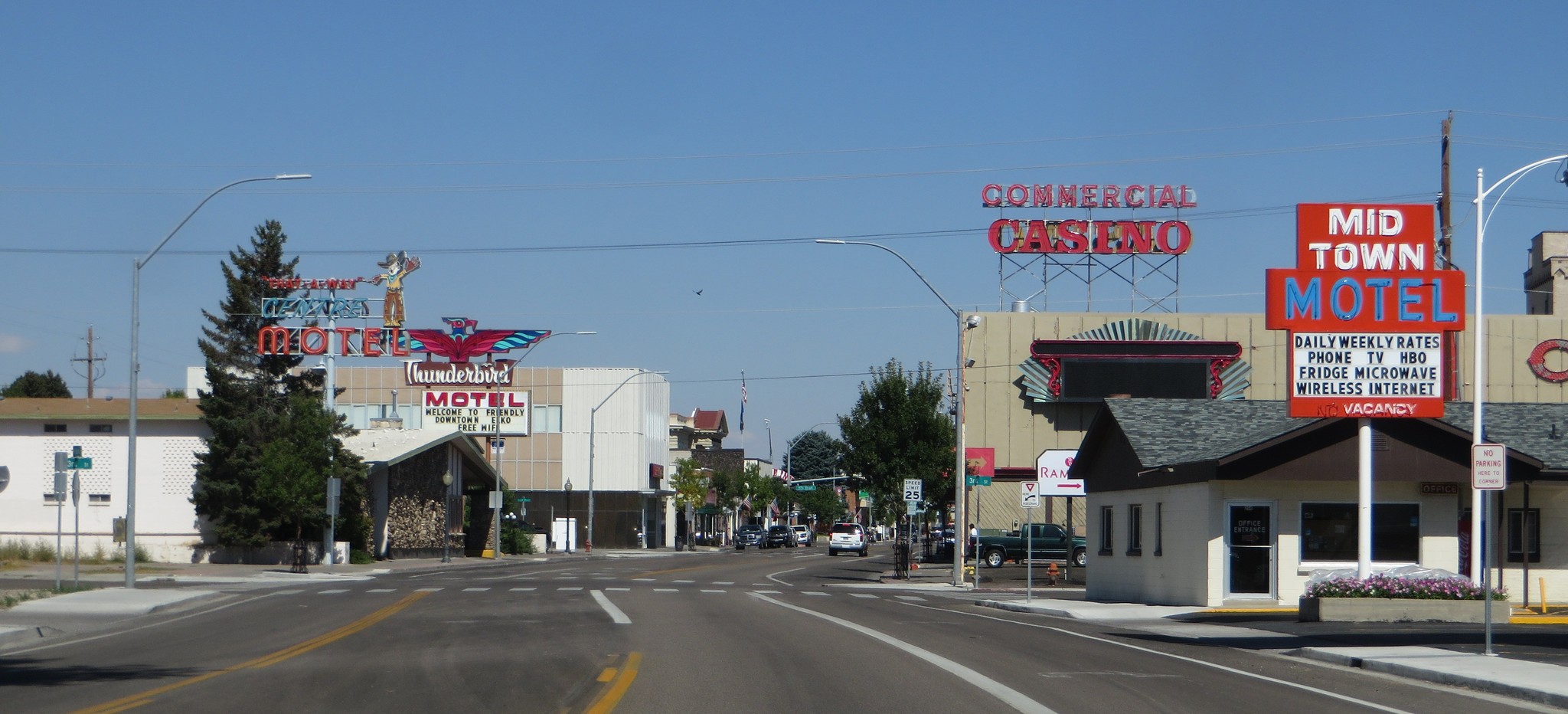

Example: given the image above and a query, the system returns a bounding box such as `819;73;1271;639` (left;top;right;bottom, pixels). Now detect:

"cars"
930;524;955;554
790;528;798;547
511;520;551;552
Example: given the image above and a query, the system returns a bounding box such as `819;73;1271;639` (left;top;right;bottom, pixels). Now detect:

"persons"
969;523;977;537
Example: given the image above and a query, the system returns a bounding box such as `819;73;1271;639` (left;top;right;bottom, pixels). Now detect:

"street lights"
564;477;572;554
495;331;598;559
816;237;981;584
441;470;453;564
588;370;670;551
124;173;314;589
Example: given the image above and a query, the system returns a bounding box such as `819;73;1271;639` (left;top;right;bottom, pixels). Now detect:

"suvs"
791;524;812;547
894;524;919;542
767;525;793;549
828;522;871;557
733;524;768;550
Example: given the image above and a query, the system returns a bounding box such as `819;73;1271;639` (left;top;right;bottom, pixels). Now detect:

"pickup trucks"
969;522;1087;568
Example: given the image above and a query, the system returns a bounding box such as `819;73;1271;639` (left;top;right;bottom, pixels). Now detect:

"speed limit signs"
903;478;922;501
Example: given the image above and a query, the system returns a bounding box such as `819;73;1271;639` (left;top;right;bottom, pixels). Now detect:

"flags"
744;496;751;511
770;499;780;516
858;510;862;523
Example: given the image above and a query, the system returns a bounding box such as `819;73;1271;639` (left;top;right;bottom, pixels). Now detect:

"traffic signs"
1019;481;1040;508
1035;449;1086;497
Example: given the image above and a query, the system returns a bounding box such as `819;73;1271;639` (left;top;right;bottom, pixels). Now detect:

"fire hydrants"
585;539;592;553
1046;562;1060;586
